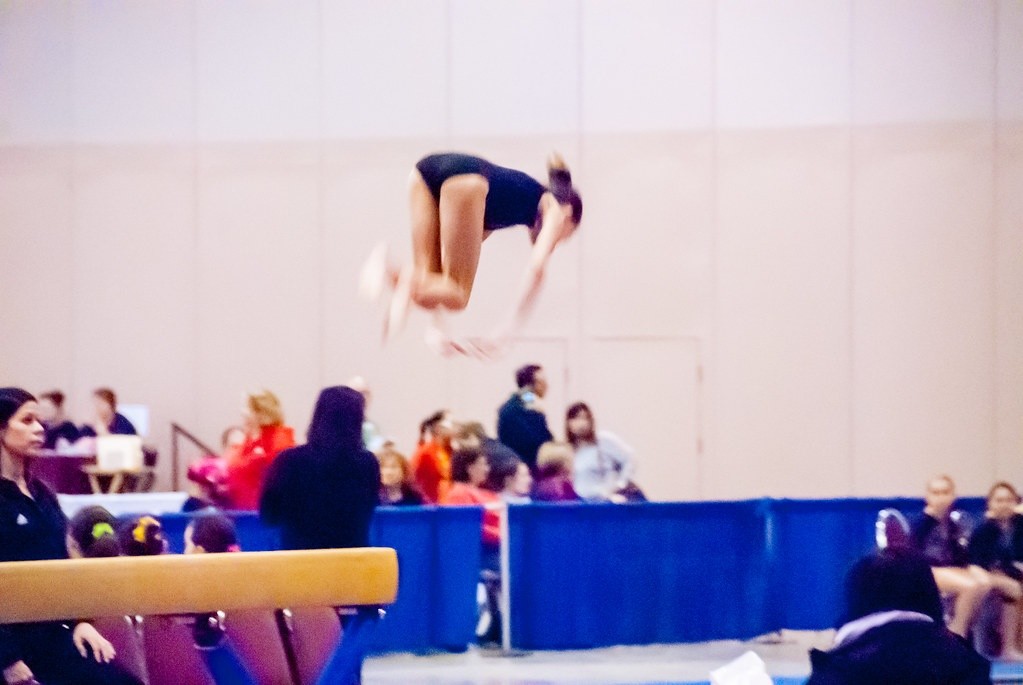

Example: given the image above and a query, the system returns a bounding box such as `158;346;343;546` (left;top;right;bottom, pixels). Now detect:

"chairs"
878;506;1023;656
86;610;345;685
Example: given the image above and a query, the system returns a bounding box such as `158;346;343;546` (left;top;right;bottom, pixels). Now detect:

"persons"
968;484;1023;660
0;387;144;685
79;390;136;437
415;411;453;500
498;364;555;463
374;447;428;506
120;515;168;555
36;392;79;448
183;515;237;552
63;506;121;558
560;402;631;502
258;385;381;551
531;440;575;502
805;547;992;685
360;152;583;361
221;392;295;511
449;451;502;570
490;456;533;500
453;411;531;492
910;474;990;638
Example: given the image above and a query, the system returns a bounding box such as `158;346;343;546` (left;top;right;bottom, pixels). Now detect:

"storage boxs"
92;433;145;471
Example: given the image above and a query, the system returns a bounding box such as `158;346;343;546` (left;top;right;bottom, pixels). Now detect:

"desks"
82;467;158;491
30;454;157;492
